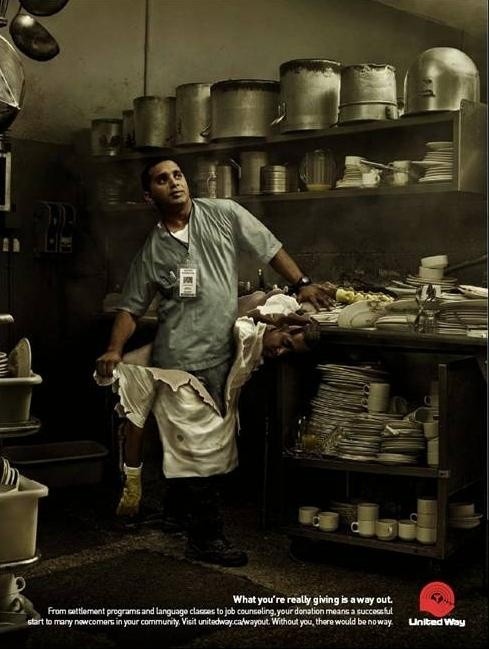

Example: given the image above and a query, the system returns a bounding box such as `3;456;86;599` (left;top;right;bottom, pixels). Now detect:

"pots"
260;164;291;194
189;153;238;198
9;13;61;63
268;58;341;135
402;44;481;120
200;78;278;143
328;63;398;127
122;110;134;152
91;118;122;157
175;82;210;148
230;150;269;198
133;95;177;149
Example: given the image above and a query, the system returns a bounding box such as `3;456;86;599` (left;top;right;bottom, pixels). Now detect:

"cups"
393;518;416;543
361;381;391;414
349;518;377;540
407;382;439;467
356;501;379;520
414;515;438;531
361;173;381;189
297;505;319;525
313;510;339;534
416;497;438;516
386;158;410;184
1;571;28;613
413;526;440;547
375;518;399;541
299;148;332;194
450;501;476;515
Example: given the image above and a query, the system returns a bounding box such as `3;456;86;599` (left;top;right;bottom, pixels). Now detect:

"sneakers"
114;477;143;519
186;537;247;567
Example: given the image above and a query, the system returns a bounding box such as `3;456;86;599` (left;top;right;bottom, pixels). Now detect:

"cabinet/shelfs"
65;100;487;212
270;328;487;578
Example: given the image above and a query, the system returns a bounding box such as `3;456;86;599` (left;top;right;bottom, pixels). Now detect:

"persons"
93;157;336;565
114;294;321;516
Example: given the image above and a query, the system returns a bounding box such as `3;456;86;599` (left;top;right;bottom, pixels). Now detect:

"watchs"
294;275;311;290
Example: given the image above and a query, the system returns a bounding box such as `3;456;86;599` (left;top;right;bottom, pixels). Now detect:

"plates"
410;140;456;183
299;363;426;465
309;254;486;340
1;458;22;494
337;298;382;328
454;515;483;530
335;156;367;190
0;347;10;376
12;334;34;378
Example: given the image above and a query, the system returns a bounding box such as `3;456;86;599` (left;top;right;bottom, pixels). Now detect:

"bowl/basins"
419;255;449;279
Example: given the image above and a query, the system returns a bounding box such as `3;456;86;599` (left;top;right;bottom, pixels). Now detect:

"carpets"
0;495;489;649
